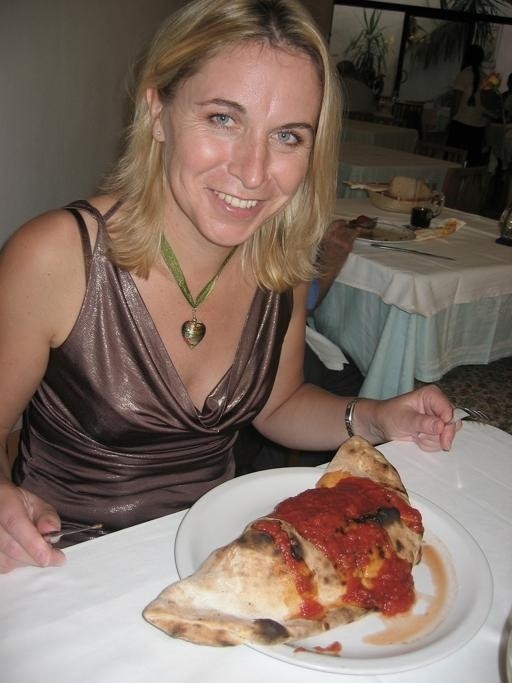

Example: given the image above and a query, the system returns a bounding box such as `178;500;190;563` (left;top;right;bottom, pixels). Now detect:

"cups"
410;176;446;229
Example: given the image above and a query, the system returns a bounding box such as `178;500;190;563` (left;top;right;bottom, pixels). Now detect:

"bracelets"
344;395;367;438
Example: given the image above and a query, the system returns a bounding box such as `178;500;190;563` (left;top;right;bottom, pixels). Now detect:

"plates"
173;466;495;676
345;220;417;244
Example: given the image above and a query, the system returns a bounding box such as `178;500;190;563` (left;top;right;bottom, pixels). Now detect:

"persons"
1;0;462;576
303;216;361;393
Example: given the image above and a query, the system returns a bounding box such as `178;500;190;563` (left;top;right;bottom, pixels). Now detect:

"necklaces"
154;236;243;350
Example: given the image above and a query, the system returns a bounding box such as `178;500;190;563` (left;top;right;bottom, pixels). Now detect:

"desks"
334;119;465;199
308;199;511;401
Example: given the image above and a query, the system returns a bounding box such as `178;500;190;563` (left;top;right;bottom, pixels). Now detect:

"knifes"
371;244;457;261
42;524;104;540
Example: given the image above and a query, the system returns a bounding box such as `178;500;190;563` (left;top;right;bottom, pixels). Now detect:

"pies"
143;435;424;649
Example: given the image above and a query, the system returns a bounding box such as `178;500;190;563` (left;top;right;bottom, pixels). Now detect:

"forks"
445;406;492;427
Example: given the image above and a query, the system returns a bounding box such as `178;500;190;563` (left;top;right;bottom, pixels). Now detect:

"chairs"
386;101;423;130
